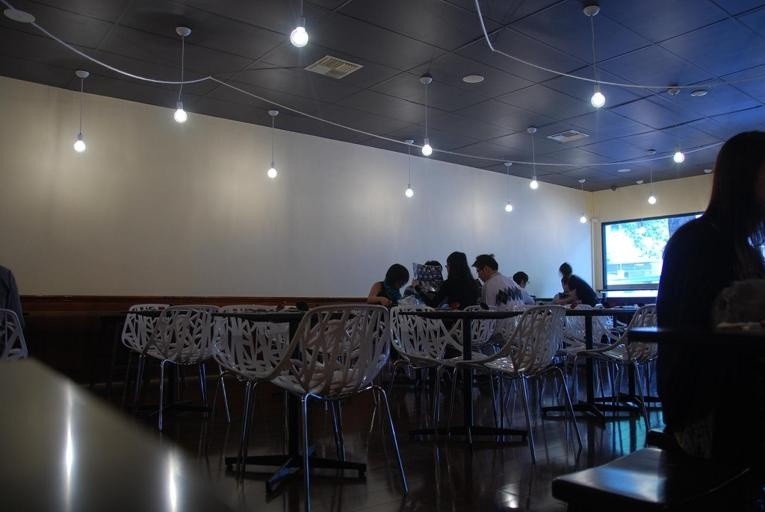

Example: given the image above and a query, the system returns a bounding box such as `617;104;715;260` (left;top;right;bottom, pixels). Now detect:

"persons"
655;129;764;511
366;251;598;396
